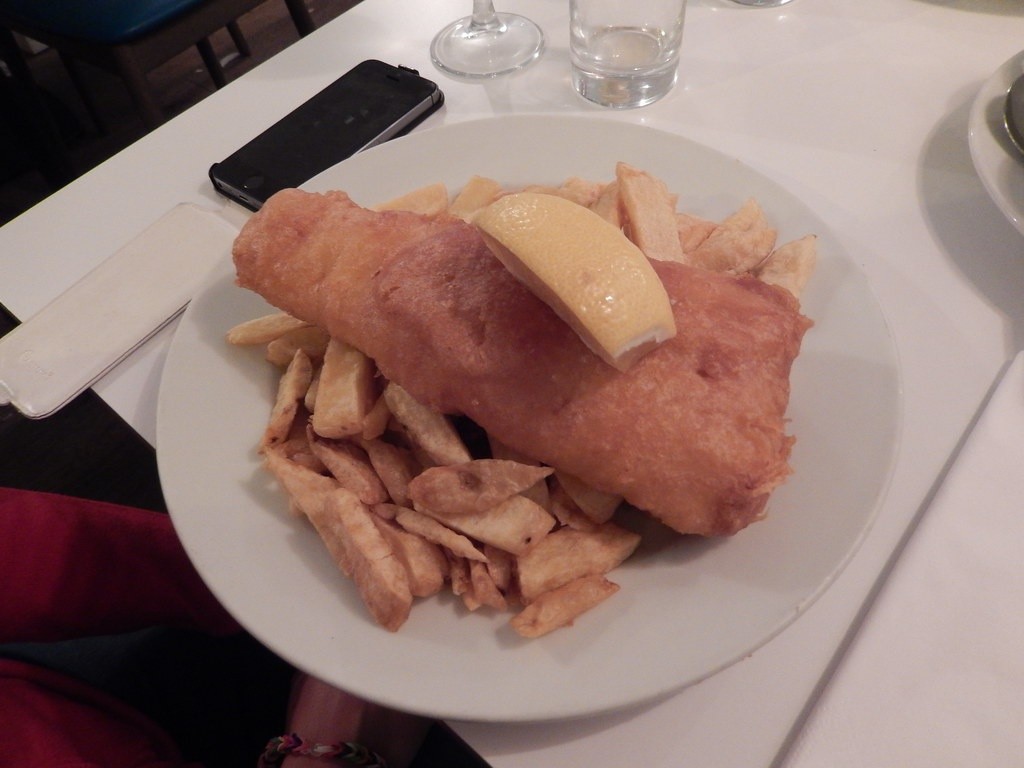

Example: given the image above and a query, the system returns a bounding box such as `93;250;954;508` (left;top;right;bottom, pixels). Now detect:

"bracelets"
257;735;391;768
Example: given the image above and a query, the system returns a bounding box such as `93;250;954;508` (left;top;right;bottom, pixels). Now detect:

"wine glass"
430;0;545;80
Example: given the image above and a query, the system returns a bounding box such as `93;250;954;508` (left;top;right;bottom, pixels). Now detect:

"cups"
568;0;687;111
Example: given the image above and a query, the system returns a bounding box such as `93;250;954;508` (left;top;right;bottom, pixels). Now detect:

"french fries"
226;160;818;644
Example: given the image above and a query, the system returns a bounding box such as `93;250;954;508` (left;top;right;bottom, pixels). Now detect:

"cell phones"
209;60;443;213
1;201;240;422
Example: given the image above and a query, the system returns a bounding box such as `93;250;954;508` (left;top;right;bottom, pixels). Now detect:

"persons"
0;485;431;768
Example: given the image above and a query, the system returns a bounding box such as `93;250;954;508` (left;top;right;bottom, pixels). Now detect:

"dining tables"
0;0;1024;767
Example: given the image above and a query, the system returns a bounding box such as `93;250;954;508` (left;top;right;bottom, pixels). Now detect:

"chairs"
0;0;317;135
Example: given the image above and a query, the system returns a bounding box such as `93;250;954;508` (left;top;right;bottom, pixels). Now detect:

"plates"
967;49;1024;238
156;111;907;722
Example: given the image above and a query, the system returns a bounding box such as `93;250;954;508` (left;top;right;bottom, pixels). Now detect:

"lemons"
471;191;677;370
